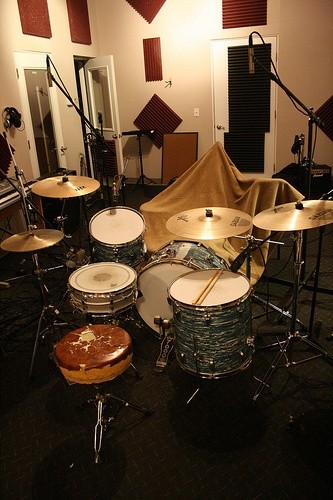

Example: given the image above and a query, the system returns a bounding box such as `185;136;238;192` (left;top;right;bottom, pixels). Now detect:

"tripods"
0;116;333;465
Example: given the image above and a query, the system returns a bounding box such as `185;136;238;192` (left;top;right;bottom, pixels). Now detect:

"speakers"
272;163;302;196
299;162;332;200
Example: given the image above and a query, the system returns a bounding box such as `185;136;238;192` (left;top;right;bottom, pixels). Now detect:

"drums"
167;269;255;378
136;241;230;337
89;206;146;267
69;262;137;318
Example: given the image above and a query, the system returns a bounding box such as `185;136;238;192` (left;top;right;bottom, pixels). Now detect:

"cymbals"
166;207;252;239
252;200;333;231
31;175;100;198
1;229;64;252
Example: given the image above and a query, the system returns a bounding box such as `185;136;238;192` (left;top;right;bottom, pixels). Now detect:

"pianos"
0;176;37;211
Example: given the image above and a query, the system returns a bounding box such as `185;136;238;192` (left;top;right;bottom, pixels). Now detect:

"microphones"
247;34;256;74
47;55;52;87
291;134;305;154
56;256;76;269
